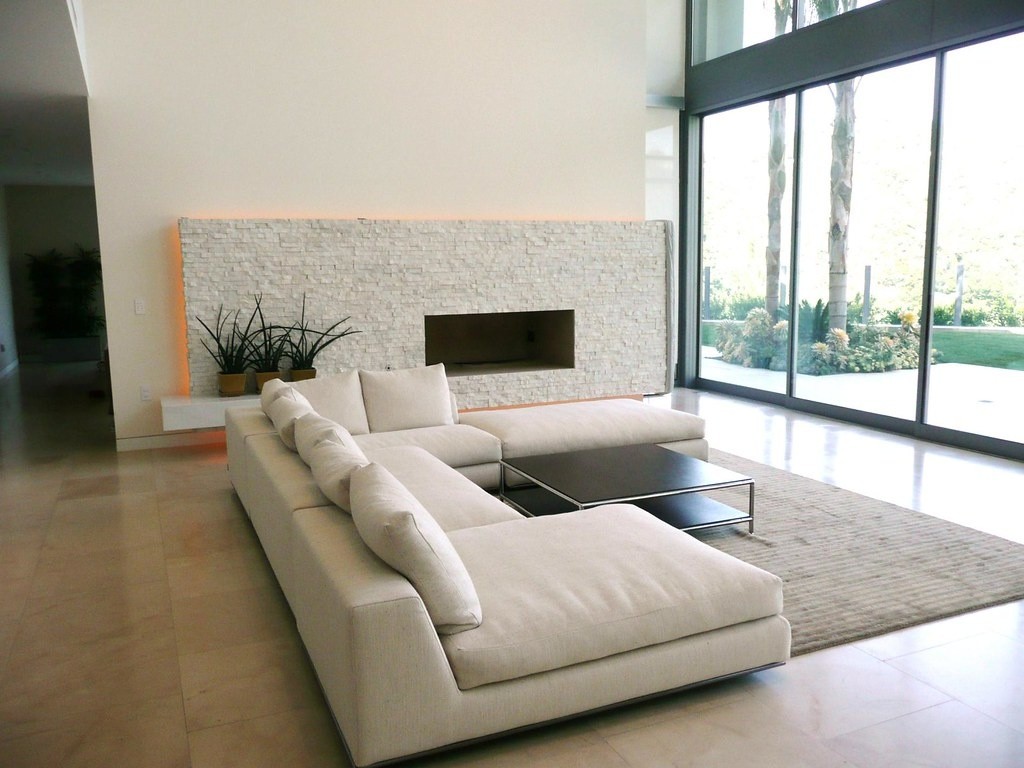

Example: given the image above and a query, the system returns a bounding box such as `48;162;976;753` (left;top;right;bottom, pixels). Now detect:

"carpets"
493;447;1024;661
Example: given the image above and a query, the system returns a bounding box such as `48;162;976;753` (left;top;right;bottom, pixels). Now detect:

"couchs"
224;361;794;768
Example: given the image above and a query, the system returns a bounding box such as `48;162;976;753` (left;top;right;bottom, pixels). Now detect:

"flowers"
713;304;941;376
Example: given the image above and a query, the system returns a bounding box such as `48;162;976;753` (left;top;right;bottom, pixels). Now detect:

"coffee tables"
499;444;755;539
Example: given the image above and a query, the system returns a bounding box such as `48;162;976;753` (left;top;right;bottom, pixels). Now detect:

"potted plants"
280;291;363;381
18;242;106;363
231;292;299;395
194;292;263;398
